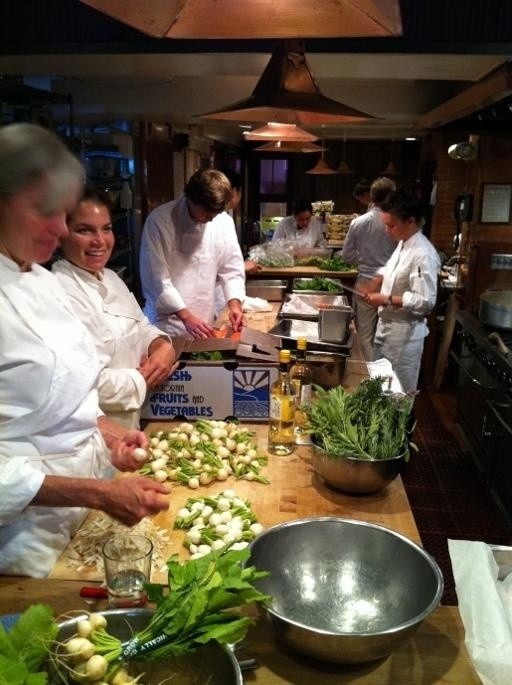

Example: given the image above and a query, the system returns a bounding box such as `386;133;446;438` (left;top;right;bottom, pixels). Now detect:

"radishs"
35;550;275;685
133;419;264;489
176;490;265;560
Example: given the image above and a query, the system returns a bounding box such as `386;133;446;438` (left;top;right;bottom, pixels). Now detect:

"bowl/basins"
248;518;446;669
51;611;244;685
307;432;406;496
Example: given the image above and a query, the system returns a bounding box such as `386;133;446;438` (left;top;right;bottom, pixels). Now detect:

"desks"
47;298;427;585
249;263;362;279
1;572;479;685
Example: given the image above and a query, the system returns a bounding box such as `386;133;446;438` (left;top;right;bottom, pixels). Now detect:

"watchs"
386;295;392;305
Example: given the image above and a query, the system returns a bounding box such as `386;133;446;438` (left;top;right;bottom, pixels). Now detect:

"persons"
272;199;326;252
224;172;263;275
0;122;172;579
138;169;247;339
357;193;442;415
352;178;370;205
50;196;176;430
340;176;397;361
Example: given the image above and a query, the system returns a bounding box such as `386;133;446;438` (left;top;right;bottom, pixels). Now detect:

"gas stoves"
453;306;512;376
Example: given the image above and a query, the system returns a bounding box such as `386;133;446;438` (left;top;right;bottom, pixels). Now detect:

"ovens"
444;332;511;525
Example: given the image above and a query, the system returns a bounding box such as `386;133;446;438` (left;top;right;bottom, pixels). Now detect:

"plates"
443;280;456;284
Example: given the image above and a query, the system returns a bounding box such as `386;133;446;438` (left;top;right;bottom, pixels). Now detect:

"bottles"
268;350;296;456
289;338;312;436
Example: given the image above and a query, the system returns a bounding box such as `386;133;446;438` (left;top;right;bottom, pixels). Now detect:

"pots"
478;287;512;329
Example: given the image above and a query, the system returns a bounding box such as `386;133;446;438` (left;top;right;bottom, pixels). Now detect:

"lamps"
304;136;338;174
251;142;330;153
191;51;386;125
240;122;322;143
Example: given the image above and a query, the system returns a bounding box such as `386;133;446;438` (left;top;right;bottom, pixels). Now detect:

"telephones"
454;192;472;222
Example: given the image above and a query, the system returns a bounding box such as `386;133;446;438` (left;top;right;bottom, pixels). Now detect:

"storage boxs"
138;327;283;424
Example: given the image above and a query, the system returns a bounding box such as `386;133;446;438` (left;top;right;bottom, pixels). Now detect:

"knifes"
324;277;367;298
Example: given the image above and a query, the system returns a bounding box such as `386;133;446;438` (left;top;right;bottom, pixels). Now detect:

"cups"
102;535;154;609
489;253;512;269
